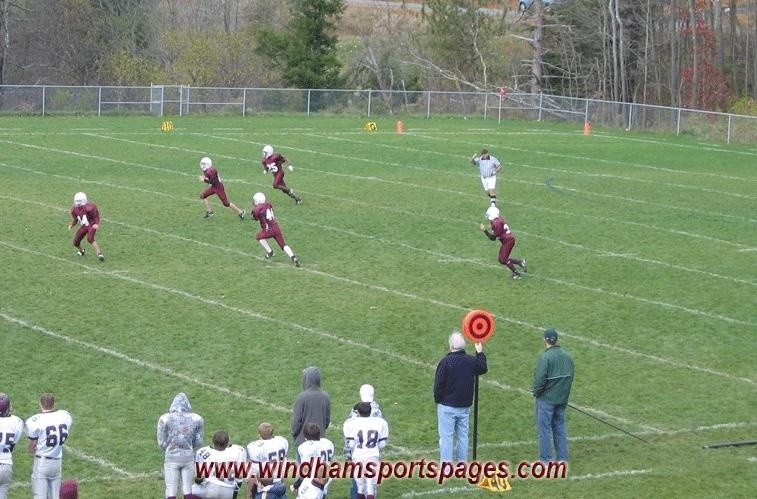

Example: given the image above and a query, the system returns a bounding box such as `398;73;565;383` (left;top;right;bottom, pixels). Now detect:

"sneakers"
73;248;87;258
202;209;216;220
96;252;105;262
511;270;522;280
520;258;528;273
293;257;301;268
287;187;305;206
238;209;247;222
263;250;275;260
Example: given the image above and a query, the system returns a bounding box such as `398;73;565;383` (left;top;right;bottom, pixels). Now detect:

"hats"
60;478;80;497
358;382;375;403
544;328;559;341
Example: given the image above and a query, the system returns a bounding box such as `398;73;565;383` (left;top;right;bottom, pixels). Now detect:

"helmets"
261;144;274;158
73;191;88;208
199;156;212;171
485;206;500;221
252;192;266;206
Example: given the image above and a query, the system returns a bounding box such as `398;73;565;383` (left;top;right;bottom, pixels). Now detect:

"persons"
59;479;78;499
26;395;72;499
157;366;389;499
472;149;502;202
479;207;526;278
68;192;104;262
198;157;245;220
251;192;300;267
261;146;303;204
433;332;488;462
0;396;24;499
532;330;575;465
